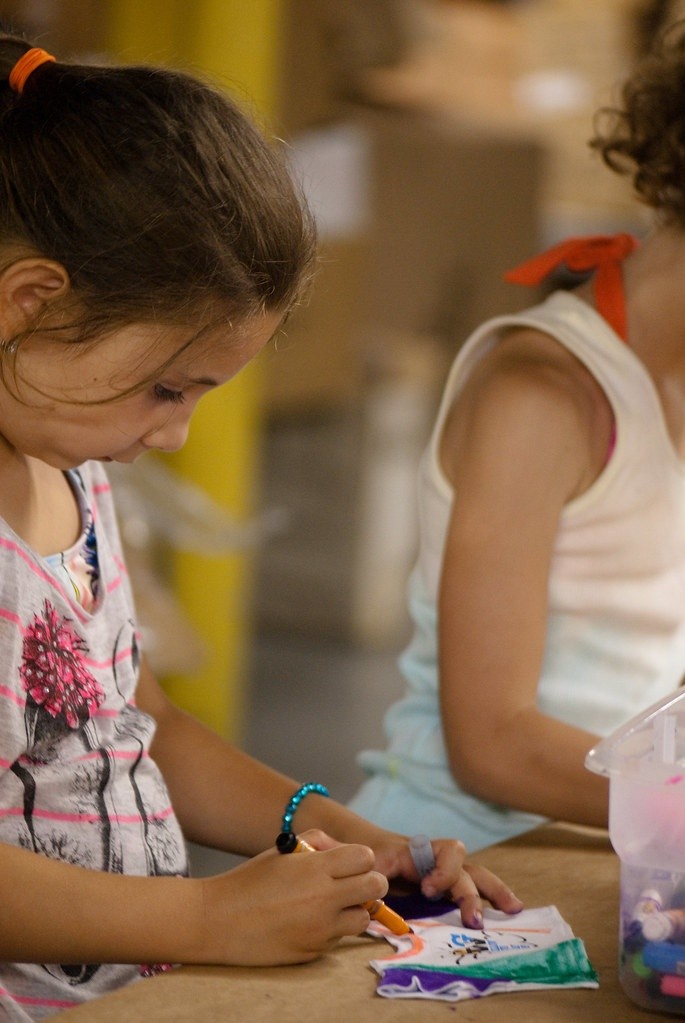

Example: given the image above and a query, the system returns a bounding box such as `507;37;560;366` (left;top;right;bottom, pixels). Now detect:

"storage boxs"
583;685;685;1017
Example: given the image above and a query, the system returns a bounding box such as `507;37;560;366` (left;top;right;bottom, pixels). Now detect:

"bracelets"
282;783;330;834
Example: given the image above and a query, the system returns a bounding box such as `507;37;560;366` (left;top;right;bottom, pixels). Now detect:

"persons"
345;18;685;848
0;33;526;1023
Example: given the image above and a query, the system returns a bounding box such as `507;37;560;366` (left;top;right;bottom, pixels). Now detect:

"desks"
40;815;685;1023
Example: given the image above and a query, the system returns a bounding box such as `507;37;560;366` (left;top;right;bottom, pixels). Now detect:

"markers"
275;831;415;936
625;887;685;999
